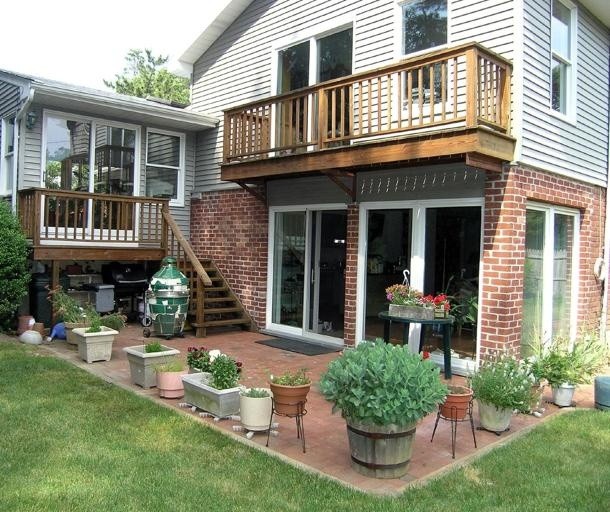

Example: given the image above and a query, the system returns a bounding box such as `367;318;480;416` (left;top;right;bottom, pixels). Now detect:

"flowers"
188;346;211;374
45;284;82;323
385;283;450;313
469;351;548;417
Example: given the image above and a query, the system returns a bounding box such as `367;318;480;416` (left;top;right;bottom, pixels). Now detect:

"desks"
380;313;455;379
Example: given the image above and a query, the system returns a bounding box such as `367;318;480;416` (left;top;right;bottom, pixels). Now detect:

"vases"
65;322;88;345
389;302;448;322
474;398;512;432
189;365;205;379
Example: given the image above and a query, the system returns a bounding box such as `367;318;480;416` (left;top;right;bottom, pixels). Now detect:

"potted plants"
321;336;446;481
437;385;472;419
270;361;313;418
530;338;606;408
182;356;246;419
238;385;272;432
124;340;180;390
74;324;118;365
